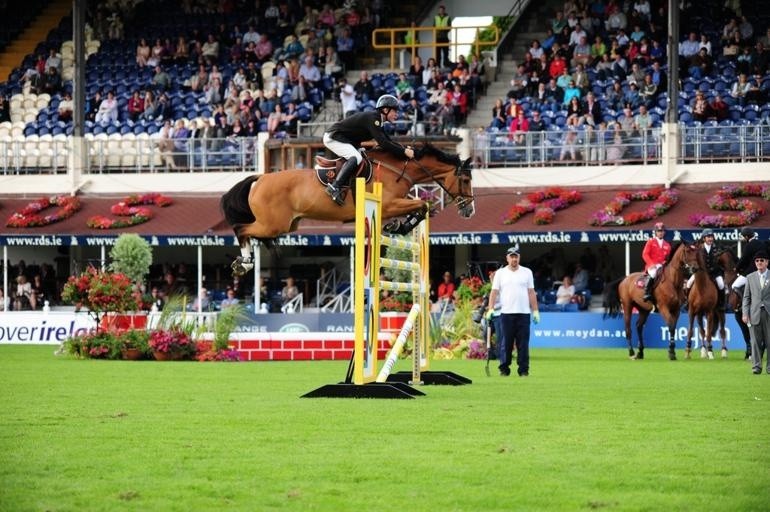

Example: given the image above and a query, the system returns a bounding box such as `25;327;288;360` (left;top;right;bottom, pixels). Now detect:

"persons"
730;222;758;311
1;255;109;311
487;245;542;376
320;94;415;207
639;221;671;302
281;277;298;314
472;269;506;371
145;263;271;313
685;227;733;315
331;6;486;137
16;2;391;173
365;271;465;312
477;1;770;169
528;247;620;311
738;250;770;377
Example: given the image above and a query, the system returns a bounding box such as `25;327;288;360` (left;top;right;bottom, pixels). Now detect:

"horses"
602;236;699;361
721;244;767;360
218;140;476;277
685;249;728;361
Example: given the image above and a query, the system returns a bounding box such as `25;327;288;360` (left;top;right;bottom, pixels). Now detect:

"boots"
325;156;359;204
642;277;655;302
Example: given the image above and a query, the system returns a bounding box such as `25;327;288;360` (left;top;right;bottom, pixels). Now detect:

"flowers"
588;187;680;226
687;182;770;227
396;277;508;359
497;187;582;227
5;196;81;231
54;330;250;363
87;191;173;230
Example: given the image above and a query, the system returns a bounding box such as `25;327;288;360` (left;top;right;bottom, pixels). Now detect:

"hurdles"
301;178;474;398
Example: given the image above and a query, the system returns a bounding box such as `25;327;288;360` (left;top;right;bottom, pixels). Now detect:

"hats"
506;247;521;256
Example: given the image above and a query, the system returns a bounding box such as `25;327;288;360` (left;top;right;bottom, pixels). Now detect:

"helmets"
702;228;714;237
654;222;665;231
375;94;400;109
741;226;755;236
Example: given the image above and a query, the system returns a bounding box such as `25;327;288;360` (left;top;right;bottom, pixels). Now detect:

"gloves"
533;310;541;325
485;308;495;321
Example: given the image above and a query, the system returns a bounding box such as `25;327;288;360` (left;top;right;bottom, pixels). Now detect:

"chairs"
0;247;770;316
0;2;769;188
